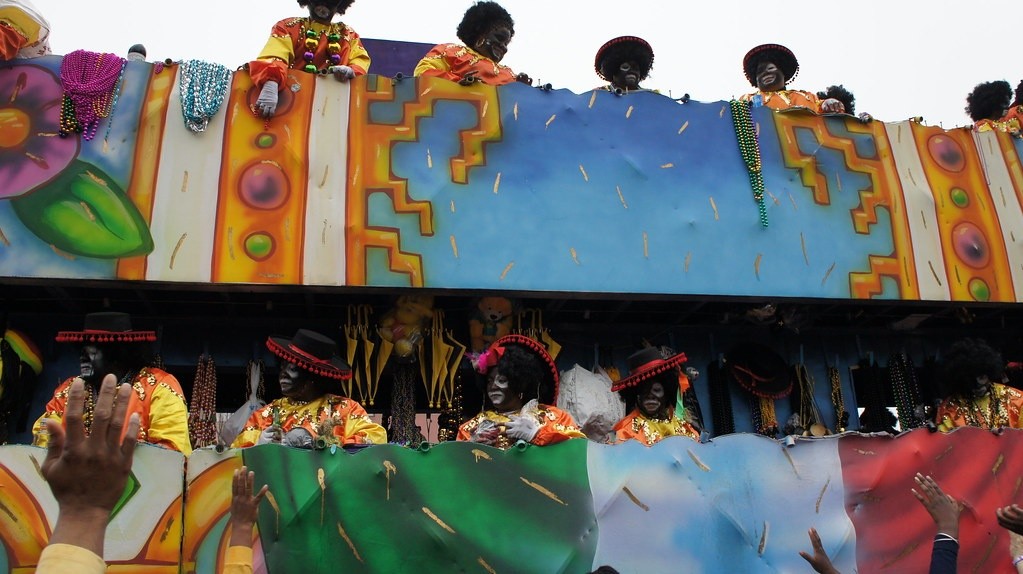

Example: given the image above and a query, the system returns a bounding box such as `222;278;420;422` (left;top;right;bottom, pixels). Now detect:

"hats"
266;329;352;380
611;346;687;392
470;333;559;408
56;312;156;341
594;36;654;82
744;43;798;84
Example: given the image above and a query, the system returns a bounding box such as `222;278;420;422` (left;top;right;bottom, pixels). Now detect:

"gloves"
255;81;278;117
329;64;356;81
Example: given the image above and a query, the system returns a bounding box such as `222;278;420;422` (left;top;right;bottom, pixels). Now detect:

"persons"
0;373;139;573
964;79;1023;122
249;0;371;118
414;0;517;82
935;364;1023;432
230;328;387;448
580;347;700;444
739;44;856;115
224;465;268;574
456;334;587;448
799;471;1023;574
595;36;654;92
31;312;192;455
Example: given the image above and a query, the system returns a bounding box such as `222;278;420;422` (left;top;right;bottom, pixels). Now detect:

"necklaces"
291;400;307;406
301;30;341;74
494;409;518;415
888;353;921;431
84;384;96;433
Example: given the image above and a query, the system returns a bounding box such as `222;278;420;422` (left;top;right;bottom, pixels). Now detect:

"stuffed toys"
380;293;433;356
470;296;512;350
